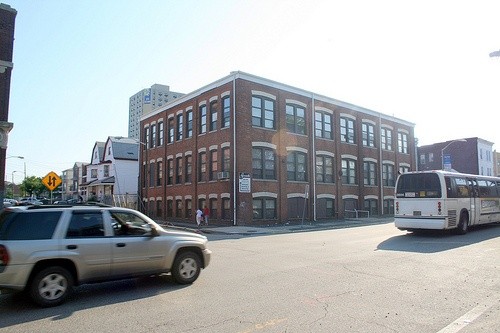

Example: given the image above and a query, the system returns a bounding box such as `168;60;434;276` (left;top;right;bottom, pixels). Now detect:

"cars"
53;198;77;205
2;196;45;206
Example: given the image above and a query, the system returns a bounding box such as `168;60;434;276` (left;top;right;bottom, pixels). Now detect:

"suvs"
0;205;212;306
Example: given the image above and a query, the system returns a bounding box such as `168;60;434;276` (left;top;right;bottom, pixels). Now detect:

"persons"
200;213;204;225
202;205;210;226
88;192;99;203
195;208;202;226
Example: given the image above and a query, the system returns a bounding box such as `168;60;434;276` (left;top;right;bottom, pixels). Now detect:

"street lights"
441;139;468;169
12;170;16;200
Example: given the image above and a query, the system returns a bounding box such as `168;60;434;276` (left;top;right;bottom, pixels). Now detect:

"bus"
393;169;500;235
